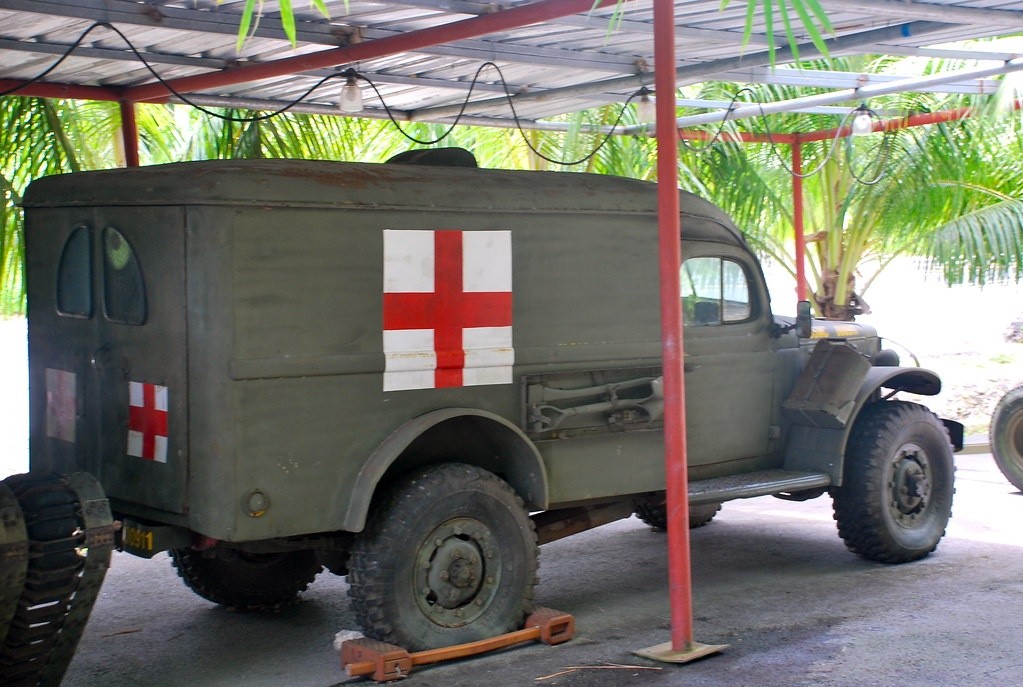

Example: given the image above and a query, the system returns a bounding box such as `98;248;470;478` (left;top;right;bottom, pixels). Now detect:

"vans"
25;147;968;651
